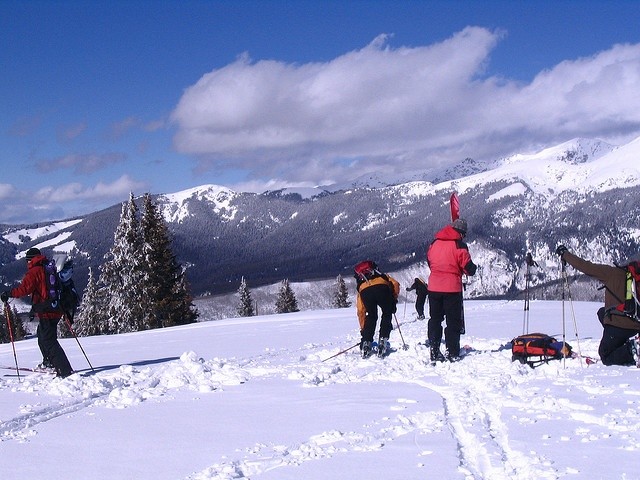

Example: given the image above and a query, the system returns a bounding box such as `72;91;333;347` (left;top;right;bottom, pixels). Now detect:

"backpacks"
512;333;572;369
353;260;379;283
618;266;640;322
44;254;80;311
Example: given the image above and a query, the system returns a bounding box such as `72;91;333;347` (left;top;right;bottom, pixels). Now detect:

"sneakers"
629;333;640;368
56;368;73;376
418;315;425;320
38;355;55;368
377;342;390;352
431;349;445;359
449;349;467;362
360;342;373;352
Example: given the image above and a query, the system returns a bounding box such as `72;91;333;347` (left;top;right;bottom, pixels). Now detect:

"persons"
355;272;401;356
0;248;75;380
555;244;640;368
427;218;478;364
407;278;429;321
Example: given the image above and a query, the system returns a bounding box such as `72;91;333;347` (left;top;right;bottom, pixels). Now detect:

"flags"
449;190;460;223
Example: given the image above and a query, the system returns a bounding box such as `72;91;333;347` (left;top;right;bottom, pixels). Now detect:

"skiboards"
0;364;89;379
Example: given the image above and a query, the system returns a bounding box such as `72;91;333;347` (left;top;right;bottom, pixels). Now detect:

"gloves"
406;287;411;291
555;244;568;256
1;289;13;302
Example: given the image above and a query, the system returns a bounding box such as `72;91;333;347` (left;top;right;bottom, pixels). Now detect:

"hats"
415;278;419;282
450;219;467;235
26;248;41;261
